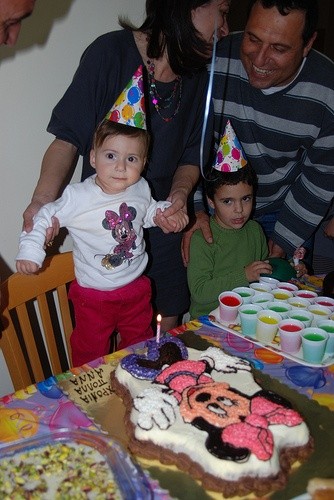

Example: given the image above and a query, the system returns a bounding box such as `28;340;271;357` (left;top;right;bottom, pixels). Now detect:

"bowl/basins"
0;429;156;499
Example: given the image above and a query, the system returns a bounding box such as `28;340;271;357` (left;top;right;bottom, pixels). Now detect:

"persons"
17;64;188;362
0;0;36;46
181;0;334;327
24;0;229;337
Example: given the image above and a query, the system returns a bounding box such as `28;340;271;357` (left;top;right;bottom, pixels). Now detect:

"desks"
0;274;334;500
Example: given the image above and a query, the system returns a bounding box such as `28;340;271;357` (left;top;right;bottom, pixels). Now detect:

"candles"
156;314;161;343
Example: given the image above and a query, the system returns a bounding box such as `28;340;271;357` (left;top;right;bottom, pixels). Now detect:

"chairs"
0;252;116;392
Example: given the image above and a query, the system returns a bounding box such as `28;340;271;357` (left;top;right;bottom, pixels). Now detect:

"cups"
219;275;334;363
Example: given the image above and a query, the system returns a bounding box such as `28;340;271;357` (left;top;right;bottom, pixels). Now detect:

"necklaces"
145;55;183;120
